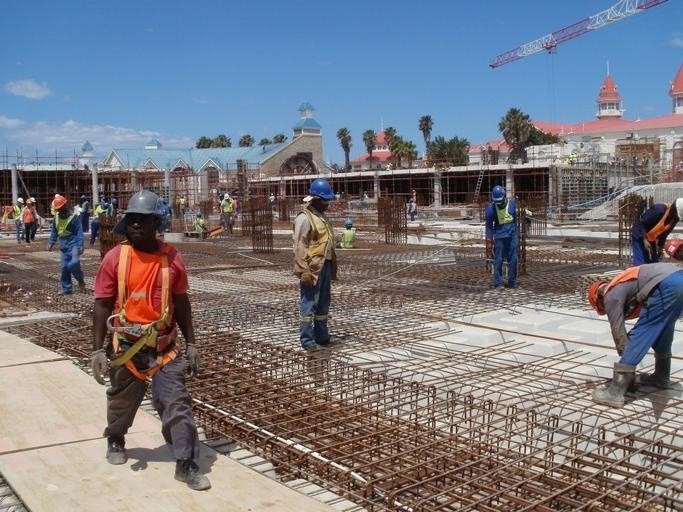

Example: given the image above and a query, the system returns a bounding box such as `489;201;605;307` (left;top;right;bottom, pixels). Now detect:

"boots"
592;362;635;409
639;353;671;386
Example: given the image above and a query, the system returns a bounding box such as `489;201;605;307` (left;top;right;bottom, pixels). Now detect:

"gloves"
91;348;110;386
184;341;200;380
300;271;314;287
485;239;494;260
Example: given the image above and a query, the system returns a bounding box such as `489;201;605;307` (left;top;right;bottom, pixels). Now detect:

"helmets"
308;178;335;200
588;279;611;315
664;238;682;259
102;203;108;209
53;196;67;210
224;194;228;200
491;185;505;201
121;189;170;217
17;197;36;204
675;197;682;222
80;195;86;199
345;220;351;225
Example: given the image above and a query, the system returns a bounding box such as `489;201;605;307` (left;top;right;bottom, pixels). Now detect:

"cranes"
489;0;669;68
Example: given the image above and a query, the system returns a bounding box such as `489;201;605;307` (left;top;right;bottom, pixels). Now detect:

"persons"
91;189;212;491
335;191;345;201
662;238;683;263
11;192;241;246
335;220;355;249
47;195;87;296
631;197;683;267
485;186;521;290
587;261;683;408
520;204;533;238
293;177;340;352
407;189;417;221
269;192;276;205
569;152;578;165
385;161;394;171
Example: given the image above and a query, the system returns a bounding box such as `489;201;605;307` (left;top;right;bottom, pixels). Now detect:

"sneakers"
173;459;210;491
106;435;126;465
